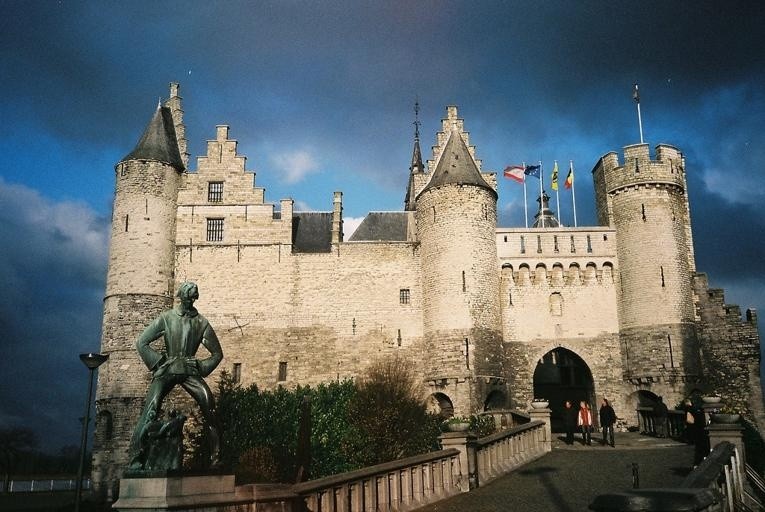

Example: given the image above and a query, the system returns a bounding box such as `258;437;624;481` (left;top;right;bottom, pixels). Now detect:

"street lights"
72;353;108;512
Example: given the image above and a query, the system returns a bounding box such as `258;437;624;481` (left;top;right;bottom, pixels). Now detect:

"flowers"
710;404;739;414
532;398;549;402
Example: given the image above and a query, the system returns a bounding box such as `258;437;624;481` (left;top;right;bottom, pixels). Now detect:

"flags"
551;162;559;190
524;164;540;179
504;165;525;184
564;163;574;190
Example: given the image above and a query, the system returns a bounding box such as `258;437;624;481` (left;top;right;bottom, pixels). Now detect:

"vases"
712;414;740;424
530;402;550;409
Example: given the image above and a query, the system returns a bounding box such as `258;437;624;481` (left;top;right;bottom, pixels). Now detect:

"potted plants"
446;417;470;431
701;392;721;403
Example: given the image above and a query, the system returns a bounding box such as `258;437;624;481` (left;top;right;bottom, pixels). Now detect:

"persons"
564;401;578;444
127;282;223;466
600;399;616;447
577;400;592;446
